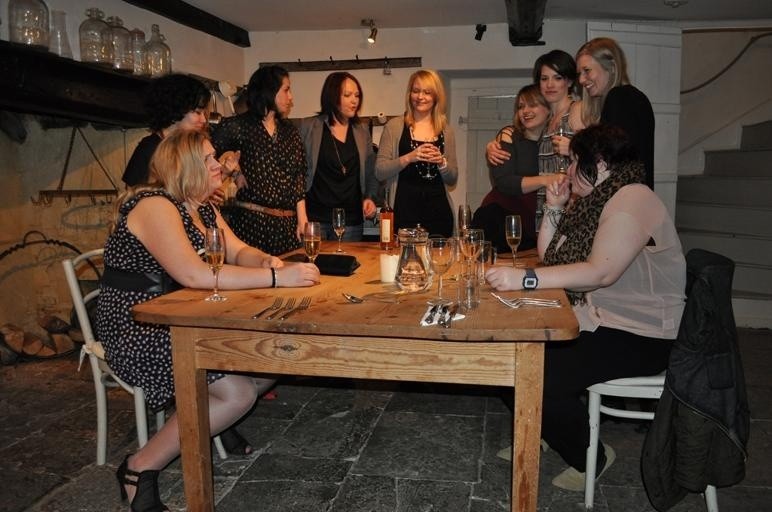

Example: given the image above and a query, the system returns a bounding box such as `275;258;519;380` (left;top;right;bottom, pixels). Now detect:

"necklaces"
548;108;565;127
327;121;356;176
409;124;445;181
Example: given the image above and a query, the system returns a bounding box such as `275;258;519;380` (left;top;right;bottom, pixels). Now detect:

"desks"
131;241;581;512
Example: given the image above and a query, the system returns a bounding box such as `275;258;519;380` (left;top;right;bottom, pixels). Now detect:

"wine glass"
202;229;229;302
422;137;438;179
332;208;346;253
556;123;569;167
426;204;493;311
304;221;321;263
505;215;522;268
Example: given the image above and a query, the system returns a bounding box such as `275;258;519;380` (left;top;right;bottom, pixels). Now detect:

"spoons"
342;291;407;303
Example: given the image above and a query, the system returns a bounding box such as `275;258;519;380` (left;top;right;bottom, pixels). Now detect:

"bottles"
395;224;432;292
79;8;171;80
380;187;395;250
7;0;74;60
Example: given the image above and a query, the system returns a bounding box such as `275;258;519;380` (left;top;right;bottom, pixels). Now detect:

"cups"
380;253;400;284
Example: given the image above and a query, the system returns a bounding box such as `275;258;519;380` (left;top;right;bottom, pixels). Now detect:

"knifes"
425;304;459;328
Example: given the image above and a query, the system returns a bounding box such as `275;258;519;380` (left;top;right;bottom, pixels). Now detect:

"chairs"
61;248;228;466
584;370;718;512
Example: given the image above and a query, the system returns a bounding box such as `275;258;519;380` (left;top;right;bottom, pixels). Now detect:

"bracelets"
542;202;567;217
436;157;447;170
270;267;278;288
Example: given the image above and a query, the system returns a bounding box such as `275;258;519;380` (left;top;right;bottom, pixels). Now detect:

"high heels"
115;454;168;511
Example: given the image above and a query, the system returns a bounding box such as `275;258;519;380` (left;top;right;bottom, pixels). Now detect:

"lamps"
362;21;378;43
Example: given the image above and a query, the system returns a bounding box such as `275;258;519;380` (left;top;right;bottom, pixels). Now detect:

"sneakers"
494;434;550;466
219;425;253;455
552;442;618;494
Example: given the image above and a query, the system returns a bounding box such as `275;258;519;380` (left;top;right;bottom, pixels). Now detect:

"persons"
211;65;312;258
293;72;381;242
98;129;323;512
485;121;689;491
373;68;459;248
467;82;566;253
121;72;212;192
485;48;586;239
550;36;655;193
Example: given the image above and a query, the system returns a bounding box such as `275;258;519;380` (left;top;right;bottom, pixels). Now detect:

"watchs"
522;267;538;291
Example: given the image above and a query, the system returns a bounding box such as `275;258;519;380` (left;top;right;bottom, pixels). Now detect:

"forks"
490;292;562;309
253;297;312;320
